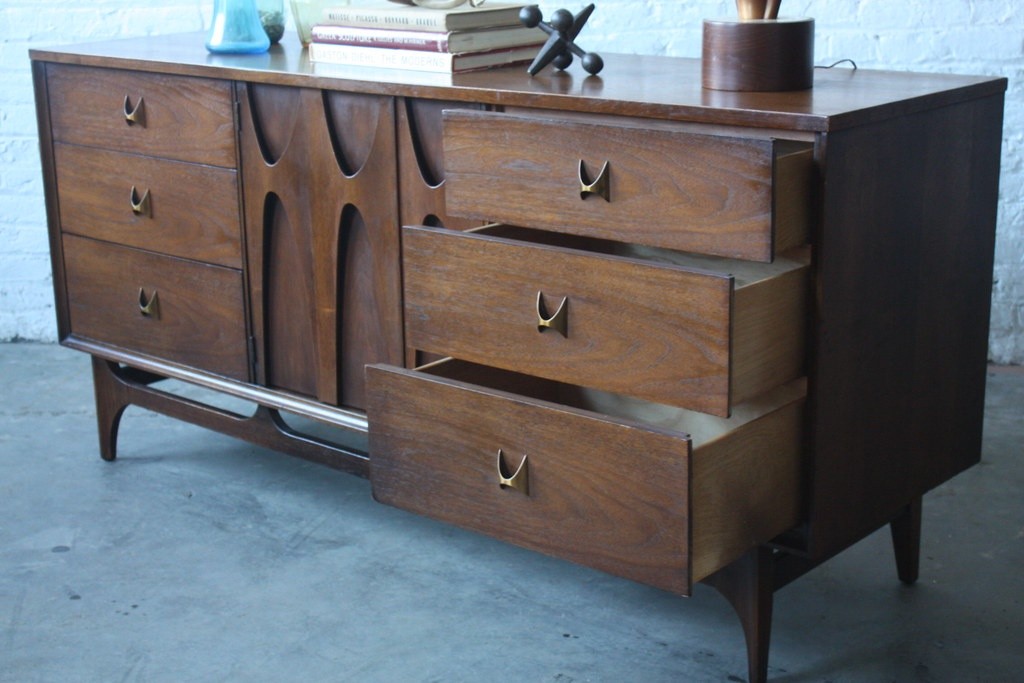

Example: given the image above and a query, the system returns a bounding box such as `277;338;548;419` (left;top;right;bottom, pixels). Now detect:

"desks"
27;29;1008;683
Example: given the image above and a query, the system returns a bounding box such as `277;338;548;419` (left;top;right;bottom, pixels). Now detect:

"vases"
205;0;271;56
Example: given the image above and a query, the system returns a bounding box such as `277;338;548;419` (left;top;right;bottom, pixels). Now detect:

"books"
310;24;555;52
308;42;551;73
325;3;538;31
